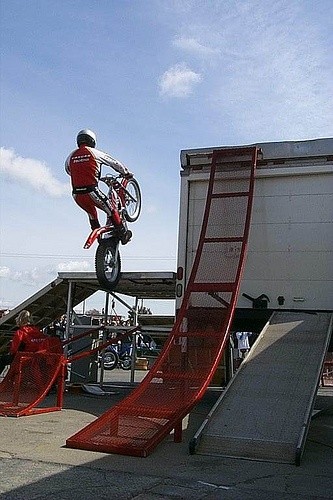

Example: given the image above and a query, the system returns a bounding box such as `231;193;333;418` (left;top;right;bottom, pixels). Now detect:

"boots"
112;222;133;245
91;222;101;243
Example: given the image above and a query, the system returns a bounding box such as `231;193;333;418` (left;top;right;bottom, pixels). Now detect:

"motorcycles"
99;341;131;370
83;173;141;290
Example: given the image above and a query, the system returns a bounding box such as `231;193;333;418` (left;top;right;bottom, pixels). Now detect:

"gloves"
125;172;134;180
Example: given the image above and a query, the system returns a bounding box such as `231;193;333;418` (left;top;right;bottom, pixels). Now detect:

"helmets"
77;129;95;148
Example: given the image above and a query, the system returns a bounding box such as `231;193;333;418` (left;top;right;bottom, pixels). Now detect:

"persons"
11;309;48;397
64;129;133;245
